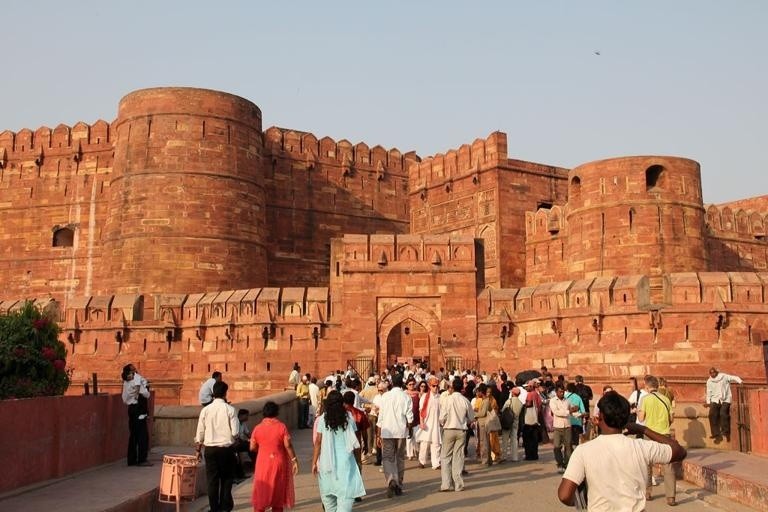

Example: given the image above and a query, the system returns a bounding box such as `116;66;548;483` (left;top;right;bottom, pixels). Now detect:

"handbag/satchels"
487;409;502;433
501;407;514;430
524;406;539;425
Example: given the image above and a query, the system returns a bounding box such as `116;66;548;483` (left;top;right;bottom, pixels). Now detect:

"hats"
368;377;375;383
509;378;541;395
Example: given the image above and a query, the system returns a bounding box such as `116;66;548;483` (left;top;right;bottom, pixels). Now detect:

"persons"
121;362;154;467
702;367;745;439
193;371;297;512
121;368;151;421
288;357;687;512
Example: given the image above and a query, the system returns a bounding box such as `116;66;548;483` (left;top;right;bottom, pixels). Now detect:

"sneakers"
557;465;566;474
646;476;675;506
720;431;728;434
387;481;402;499
137;461;153;467
138;414;147;419
710;435;718;438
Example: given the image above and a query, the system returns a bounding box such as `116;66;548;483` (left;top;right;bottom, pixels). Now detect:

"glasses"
432;385;439;387
